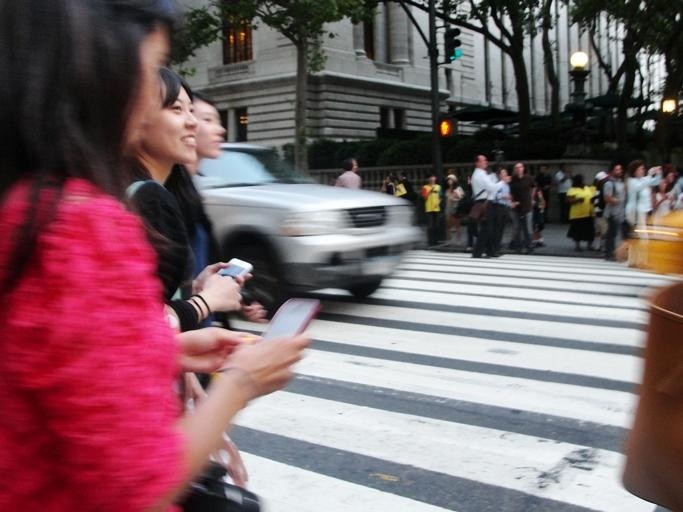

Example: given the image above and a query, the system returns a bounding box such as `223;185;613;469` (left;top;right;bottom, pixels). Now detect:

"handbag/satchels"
451;198;472;219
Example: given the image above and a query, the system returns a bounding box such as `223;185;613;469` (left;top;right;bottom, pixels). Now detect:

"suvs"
189;143;425;321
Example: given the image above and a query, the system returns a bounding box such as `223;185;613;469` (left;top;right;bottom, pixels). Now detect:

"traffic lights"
438;114;457;140
443;27;461;64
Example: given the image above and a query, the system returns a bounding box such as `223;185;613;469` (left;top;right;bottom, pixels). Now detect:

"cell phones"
217;257;254;283
256;298;321;344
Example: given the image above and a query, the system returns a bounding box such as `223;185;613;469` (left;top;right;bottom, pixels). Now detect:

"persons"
333;158;361;189
0;0;310;512
554;162;573;225
380;166;479;251
535;165;553;224
565;159;683;263
470;156;545;258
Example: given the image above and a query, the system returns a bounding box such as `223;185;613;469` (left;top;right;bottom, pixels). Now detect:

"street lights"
660;95;677;162
568;51;591;158
